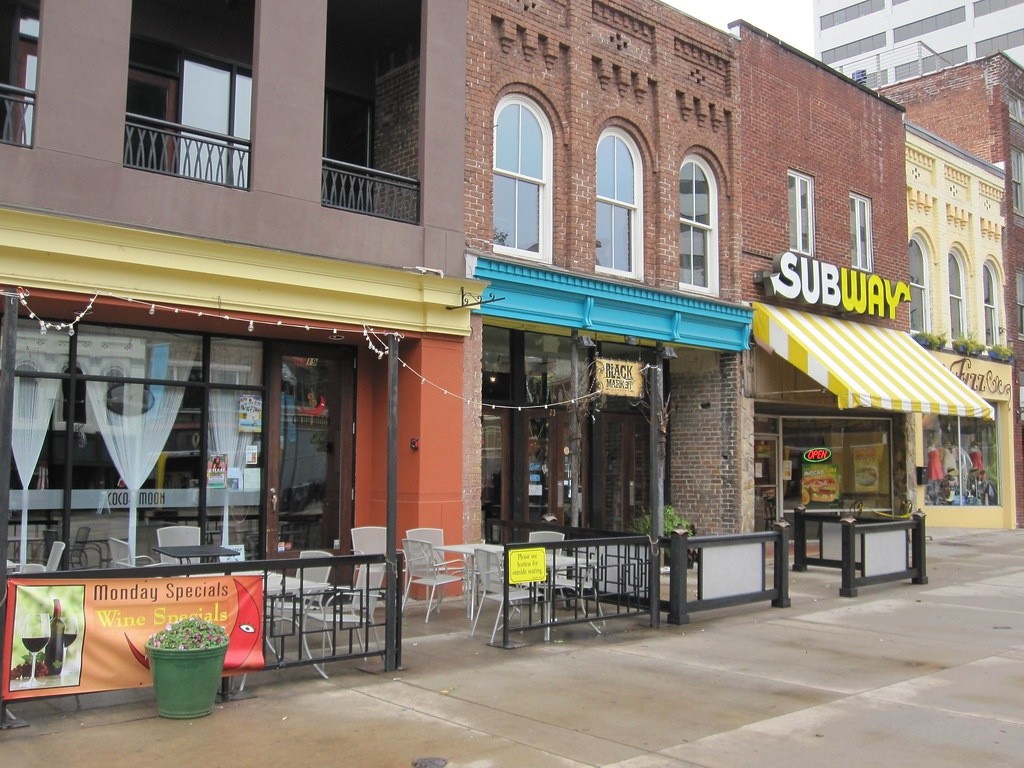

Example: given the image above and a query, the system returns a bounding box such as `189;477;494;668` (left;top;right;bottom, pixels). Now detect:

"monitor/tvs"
483;371;512;404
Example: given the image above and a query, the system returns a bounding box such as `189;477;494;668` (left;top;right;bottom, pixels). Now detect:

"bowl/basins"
856;468;877;486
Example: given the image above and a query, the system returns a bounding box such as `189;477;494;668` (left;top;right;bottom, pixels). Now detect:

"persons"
212;457;221;469
928;444;984;480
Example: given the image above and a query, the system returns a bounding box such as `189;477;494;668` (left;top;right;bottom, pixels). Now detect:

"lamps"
624;334;637;345
658;346;678;360
573;336;596;350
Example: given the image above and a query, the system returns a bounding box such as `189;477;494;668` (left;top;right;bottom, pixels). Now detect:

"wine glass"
19;613;51;687
60;614;78;675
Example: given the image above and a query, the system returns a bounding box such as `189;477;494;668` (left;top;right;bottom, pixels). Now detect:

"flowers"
9;652;63;680
148;616;229;651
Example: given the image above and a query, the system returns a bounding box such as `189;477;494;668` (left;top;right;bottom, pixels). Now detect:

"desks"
432;544;602;634
179;570;330;691
8;537;43;565
153;546;240;563
6;560;17;572
75;538;130;567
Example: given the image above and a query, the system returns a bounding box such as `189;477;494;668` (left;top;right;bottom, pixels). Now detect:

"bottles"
45;599;63;675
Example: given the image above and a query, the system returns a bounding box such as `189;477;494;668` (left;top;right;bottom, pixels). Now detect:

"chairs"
12;541;66;574
351;525;604;645
43;527;91;568
109;526;386;669
154;510;179;522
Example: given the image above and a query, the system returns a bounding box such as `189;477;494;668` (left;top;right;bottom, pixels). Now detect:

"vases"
144;641;230;719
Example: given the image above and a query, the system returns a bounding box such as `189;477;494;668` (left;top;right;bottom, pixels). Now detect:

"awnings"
752;301;994;422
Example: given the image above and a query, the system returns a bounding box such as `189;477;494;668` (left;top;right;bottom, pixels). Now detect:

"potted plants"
986;344;1017;361
951;329;986;357
911;329;948;351
